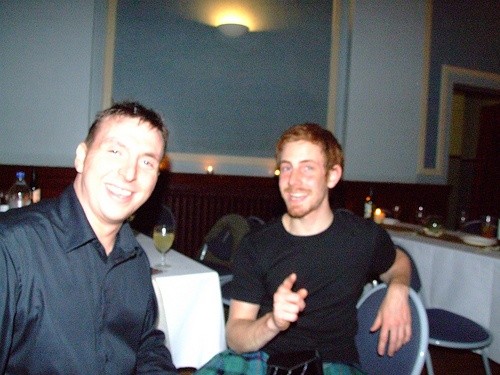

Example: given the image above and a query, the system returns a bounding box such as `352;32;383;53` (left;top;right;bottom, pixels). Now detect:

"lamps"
217;23;250;38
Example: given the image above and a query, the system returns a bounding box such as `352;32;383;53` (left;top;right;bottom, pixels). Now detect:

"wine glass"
152;207;175;270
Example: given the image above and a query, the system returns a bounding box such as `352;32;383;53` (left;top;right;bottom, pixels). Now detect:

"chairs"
128;201;497;375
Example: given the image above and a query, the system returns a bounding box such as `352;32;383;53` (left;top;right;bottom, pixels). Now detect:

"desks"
133;229;228;370
382;214;500;365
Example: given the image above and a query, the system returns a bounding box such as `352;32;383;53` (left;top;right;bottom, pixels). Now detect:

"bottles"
363;187;373;219
9;173;31;209
29;167;41;204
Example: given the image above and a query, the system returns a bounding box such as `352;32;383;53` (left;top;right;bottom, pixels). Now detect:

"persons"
224;121;413;375
0;99;186;375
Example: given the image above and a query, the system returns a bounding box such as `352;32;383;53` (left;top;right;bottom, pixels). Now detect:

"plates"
459;234;497;247
